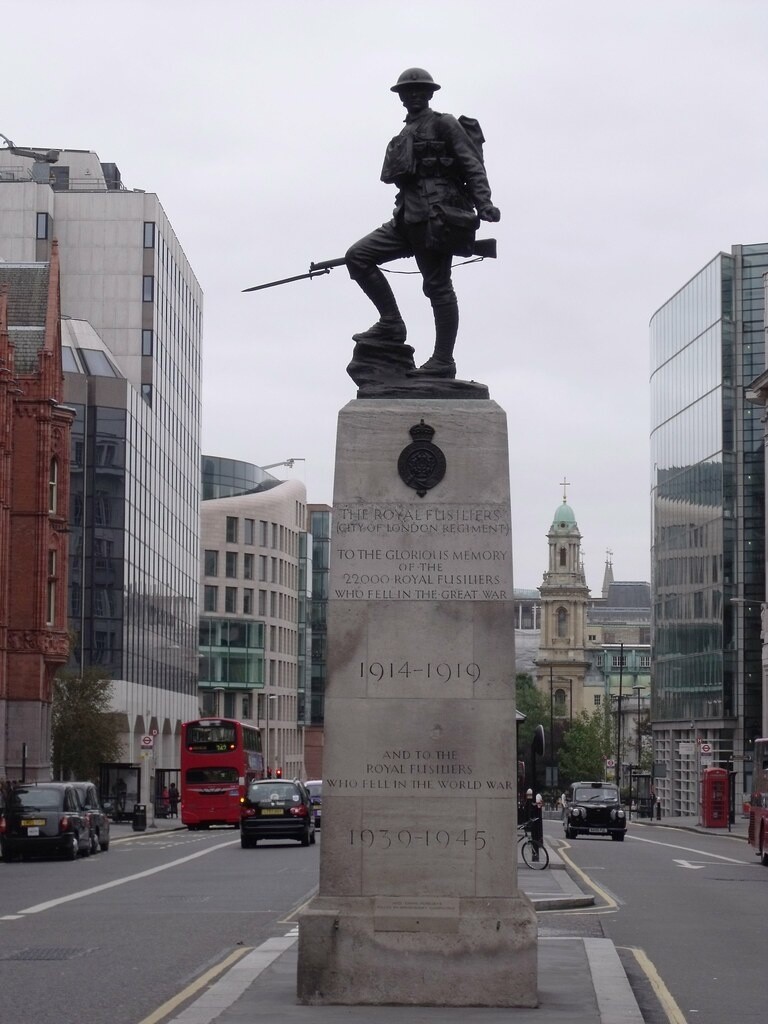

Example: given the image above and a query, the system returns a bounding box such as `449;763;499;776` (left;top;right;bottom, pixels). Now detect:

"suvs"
239;775;320;846
561;781;628;841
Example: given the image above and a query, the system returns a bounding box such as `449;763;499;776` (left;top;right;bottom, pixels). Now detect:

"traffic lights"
276;770;282;778
267;769;272;778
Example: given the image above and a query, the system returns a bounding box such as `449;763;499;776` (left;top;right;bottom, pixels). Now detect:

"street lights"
631;685;646;772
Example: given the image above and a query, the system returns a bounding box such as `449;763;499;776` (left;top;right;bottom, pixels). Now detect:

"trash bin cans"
132;804;146;832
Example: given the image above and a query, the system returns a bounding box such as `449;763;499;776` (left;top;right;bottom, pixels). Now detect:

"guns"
240;238;498;292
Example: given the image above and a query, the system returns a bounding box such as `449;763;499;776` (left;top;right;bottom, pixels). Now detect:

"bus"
180;717;266;831
746;736;768;867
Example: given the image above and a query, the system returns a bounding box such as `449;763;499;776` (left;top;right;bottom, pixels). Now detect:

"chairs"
286;788;294;798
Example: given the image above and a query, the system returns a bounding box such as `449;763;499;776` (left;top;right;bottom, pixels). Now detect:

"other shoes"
171;816;174;818
176;816;178;819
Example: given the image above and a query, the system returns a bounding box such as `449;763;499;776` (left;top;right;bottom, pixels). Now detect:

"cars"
61;782;113;853
304;780;323;824
0;782;96;860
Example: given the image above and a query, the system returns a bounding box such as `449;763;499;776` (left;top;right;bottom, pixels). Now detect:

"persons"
111;778;127;811
346;67;501;378
170;783;179;819
0;779;23;817
162;786;170;818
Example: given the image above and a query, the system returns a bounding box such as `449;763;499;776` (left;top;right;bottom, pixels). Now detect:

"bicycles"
516;817;549;871
